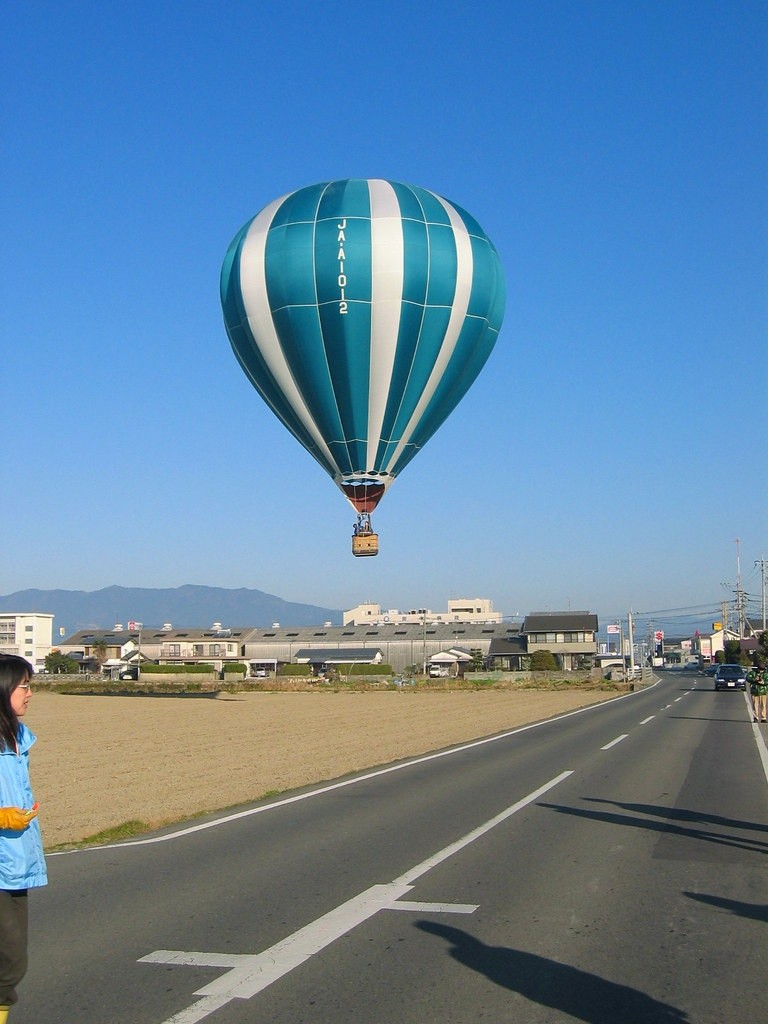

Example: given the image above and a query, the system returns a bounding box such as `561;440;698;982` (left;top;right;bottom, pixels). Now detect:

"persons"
0;654;47;1023
746;661;768;723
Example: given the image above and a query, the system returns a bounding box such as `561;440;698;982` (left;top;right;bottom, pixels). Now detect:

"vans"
430;665;446;678
251;667;271;677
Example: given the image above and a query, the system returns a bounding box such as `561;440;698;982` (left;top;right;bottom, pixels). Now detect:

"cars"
703;663;719;674
318;668;327;677
602;664;631;676
684;661;699;670
119;670;138;680
715;663;746;692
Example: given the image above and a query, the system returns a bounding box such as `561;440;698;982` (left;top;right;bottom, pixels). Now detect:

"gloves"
0;806;37;831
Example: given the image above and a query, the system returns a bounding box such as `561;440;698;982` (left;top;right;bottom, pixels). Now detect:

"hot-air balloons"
220;178;507;558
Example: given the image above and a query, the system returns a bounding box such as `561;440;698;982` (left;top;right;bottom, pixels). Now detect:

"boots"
0;1006;10;1024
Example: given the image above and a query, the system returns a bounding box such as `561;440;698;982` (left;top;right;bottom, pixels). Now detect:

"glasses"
16;684;31;691
